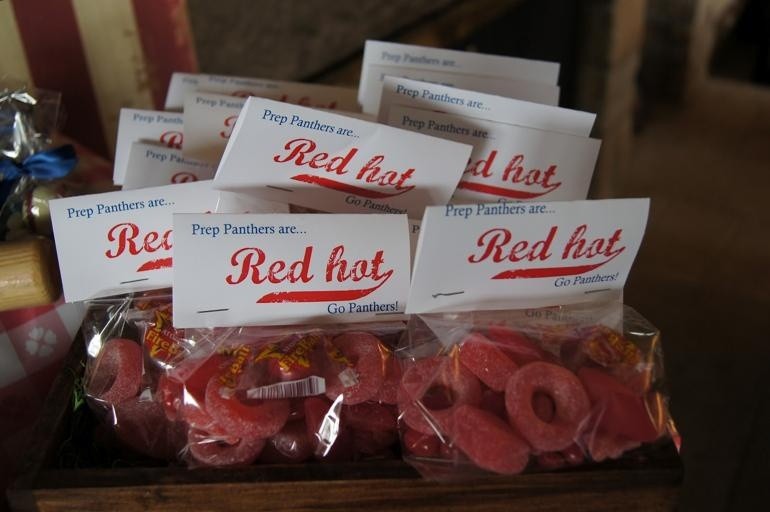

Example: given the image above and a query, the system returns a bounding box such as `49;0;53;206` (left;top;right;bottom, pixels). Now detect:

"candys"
153;330;396;481
78;288;179;455
393;321;668;476
